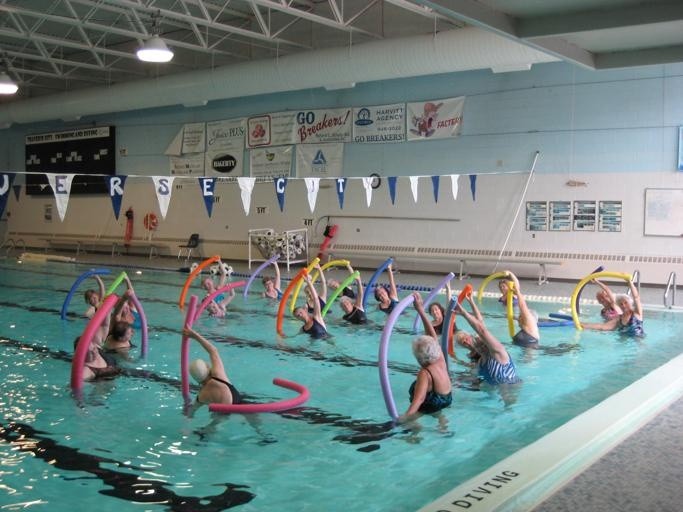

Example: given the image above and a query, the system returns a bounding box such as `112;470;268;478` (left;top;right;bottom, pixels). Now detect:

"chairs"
177;234;199;261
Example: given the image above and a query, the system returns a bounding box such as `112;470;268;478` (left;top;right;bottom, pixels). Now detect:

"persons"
592;278;623;322
577;276;645;347
263;256;538;411
189;255;243;404
73;275;136;381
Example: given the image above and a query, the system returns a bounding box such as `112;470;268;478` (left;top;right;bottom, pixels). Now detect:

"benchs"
387;251;469;280
111;240;166;260
36;236;86;255
457;252;563;286
322;247;398;275
77;237;118;256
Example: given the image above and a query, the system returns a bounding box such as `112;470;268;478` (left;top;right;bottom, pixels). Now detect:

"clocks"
369;173;381;189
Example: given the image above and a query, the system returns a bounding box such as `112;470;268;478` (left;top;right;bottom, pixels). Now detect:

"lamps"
0;67;20;96
135;9;174;66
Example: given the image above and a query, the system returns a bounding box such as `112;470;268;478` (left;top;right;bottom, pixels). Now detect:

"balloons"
576;265;605;315
570;271;634;331
536;312;575;327
